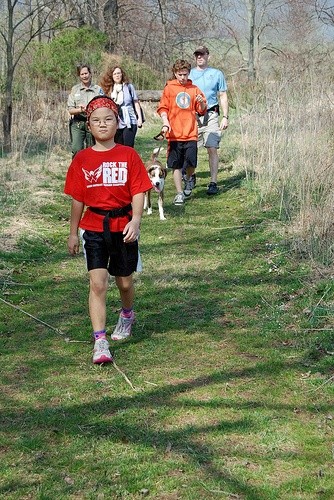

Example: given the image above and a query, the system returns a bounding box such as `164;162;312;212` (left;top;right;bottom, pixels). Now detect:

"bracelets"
223;116;228;119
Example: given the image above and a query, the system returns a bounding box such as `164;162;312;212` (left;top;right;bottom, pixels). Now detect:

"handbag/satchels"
126;82;145;123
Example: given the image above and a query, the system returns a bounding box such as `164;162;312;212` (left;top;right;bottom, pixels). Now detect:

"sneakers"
206;181;218;194
111;311;136;340
92;339;112;364
183;175;192;196
174;192;186;205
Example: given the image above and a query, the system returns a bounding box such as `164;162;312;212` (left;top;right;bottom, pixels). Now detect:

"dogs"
144;161;168;220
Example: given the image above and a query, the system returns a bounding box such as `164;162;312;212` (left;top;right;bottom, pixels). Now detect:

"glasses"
90;118;115;126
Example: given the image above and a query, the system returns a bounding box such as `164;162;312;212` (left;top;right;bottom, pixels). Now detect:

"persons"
157;59;207;204
64;95;153;363
67;65;105;159
188;47;229;194
100;65;143;148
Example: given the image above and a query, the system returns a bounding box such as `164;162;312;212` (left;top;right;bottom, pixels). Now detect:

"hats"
193;46;210;55
